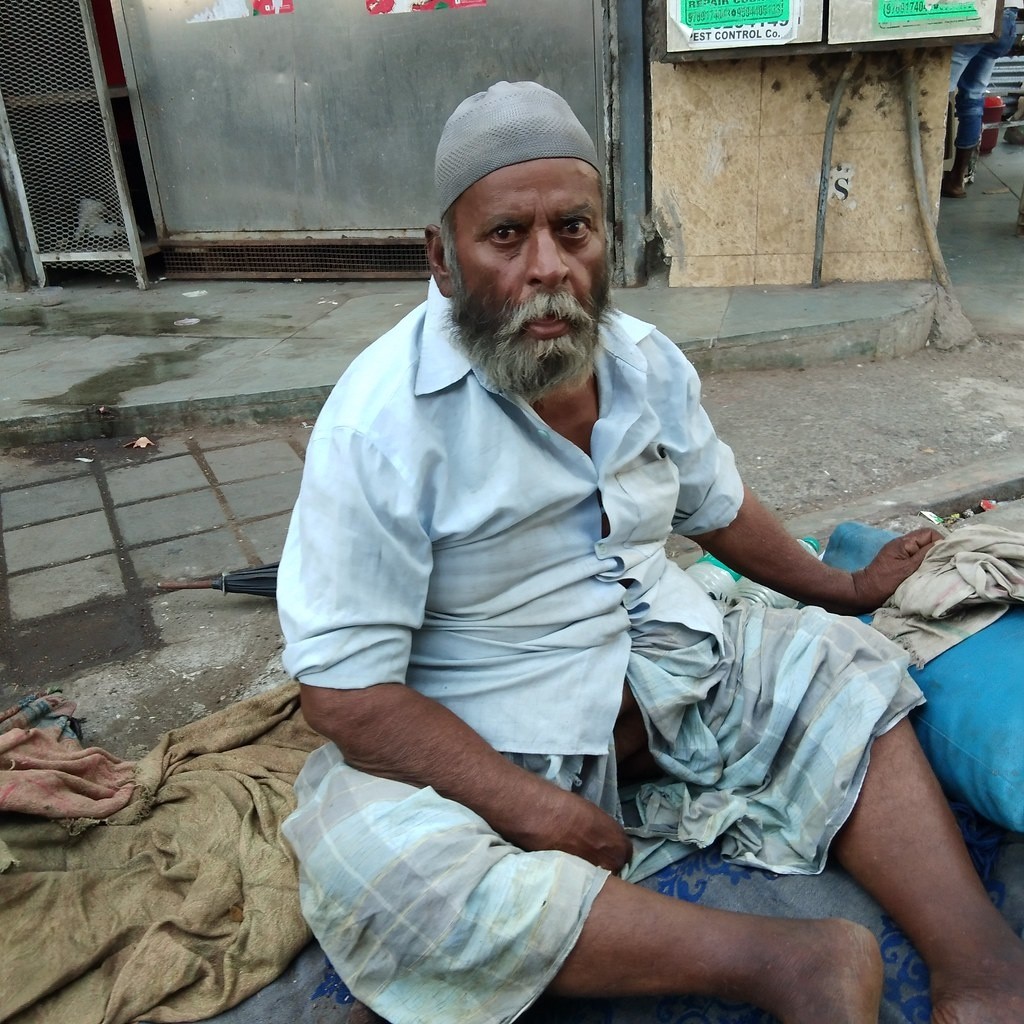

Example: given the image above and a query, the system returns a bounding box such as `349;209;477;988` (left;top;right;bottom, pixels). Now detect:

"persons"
941;0;1024;199
274;79;1024;1024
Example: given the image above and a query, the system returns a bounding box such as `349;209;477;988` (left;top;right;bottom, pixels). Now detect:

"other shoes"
939;171;968;199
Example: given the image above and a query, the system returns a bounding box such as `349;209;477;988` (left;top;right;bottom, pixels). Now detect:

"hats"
434;81;603;224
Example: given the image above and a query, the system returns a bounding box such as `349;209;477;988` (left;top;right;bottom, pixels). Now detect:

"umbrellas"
157;561;280;597
